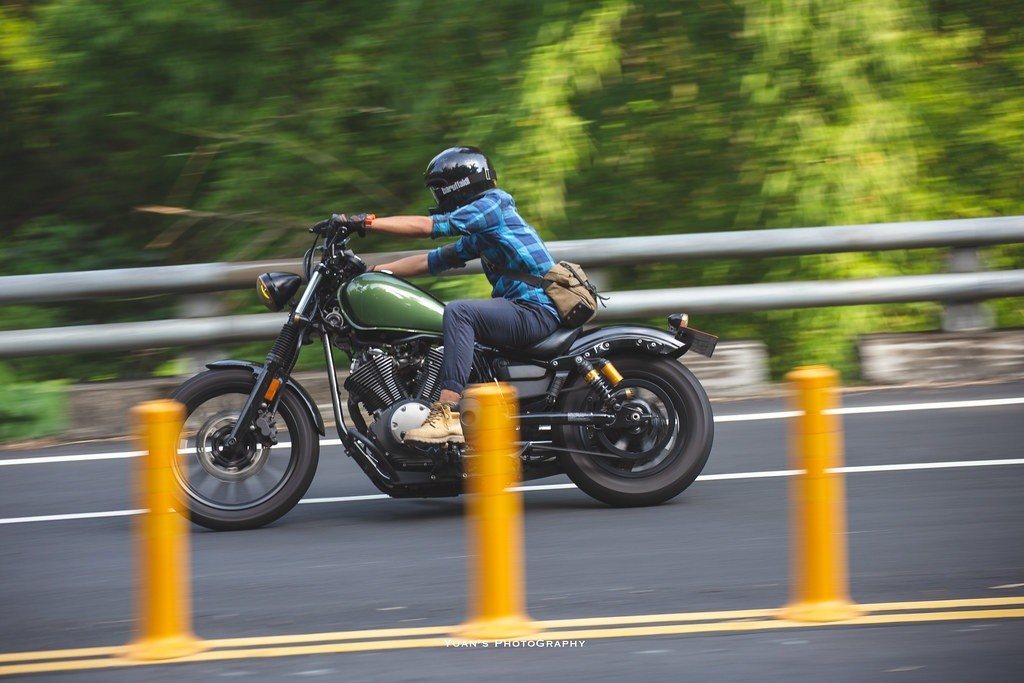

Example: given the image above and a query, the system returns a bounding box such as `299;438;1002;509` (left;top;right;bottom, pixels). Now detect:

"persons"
340;146;563;447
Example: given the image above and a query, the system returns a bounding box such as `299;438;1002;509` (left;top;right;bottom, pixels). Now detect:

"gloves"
355;256;375;273
313;213;375;246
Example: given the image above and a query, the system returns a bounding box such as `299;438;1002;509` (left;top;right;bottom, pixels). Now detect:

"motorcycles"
163;213;719;532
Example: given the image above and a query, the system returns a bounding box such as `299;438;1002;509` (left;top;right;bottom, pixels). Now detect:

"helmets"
424;147;497;216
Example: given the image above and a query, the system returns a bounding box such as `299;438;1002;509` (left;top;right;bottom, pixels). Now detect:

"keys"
345;249;361;261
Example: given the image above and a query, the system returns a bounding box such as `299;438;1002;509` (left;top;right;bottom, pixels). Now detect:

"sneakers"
403;401;465;447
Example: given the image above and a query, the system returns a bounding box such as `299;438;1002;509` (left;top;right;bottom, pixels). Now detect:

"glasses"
429;186;443;205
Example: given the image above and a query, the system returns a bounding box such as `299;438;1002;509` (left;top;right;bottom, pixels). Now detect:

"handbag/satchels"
543;260;599;328
